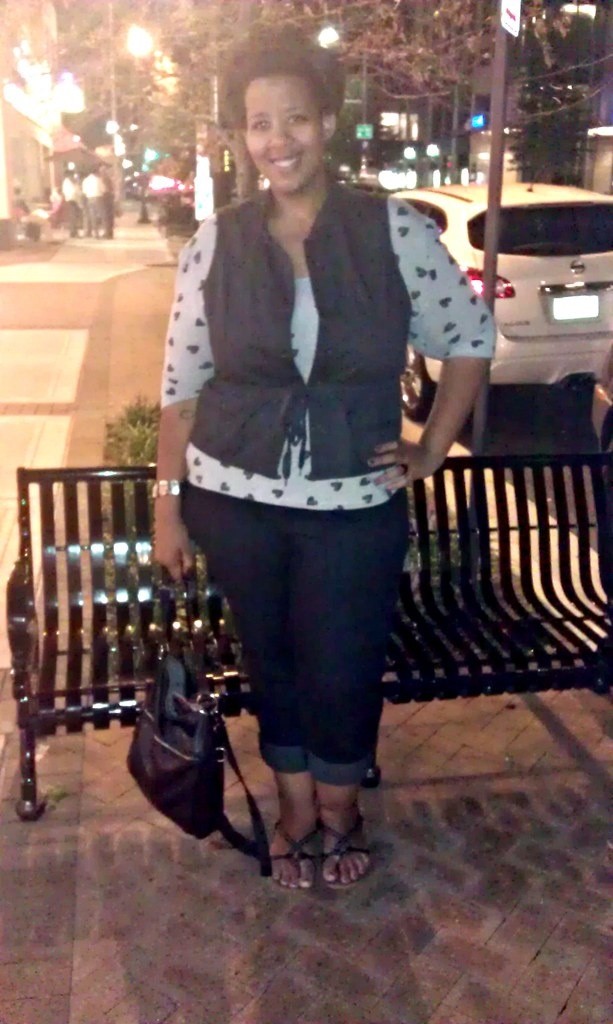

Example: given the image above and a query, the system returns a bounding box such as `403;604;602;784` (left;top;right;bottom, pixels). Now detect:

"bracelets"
152;480;183;497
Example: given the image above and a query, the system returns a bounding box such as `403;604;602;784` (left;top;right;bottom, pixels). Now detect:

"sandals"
315;804;375;895
267;813;320;897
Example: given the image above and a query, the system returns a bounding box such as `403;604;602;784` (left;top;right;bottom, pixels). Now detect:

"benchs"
6;448;613;823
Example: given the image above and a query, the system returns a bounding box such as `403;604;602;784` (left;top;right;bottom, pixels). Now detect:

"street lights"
127;24;155;227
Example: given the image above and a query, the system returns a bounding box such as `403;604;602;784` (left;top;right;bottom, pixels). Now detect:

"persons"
99;166;114;237
82;167;109;238
63;170;82;236
12;186;40;241
47;188;64;227
153;25;494;890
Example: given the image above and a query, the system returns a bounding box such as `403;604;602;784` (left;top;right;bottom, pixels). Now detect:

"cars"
387;180;613;426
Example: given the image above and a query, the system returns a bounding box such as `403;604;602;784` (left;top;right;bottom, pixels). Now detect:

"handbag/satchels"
126;547;227;840
